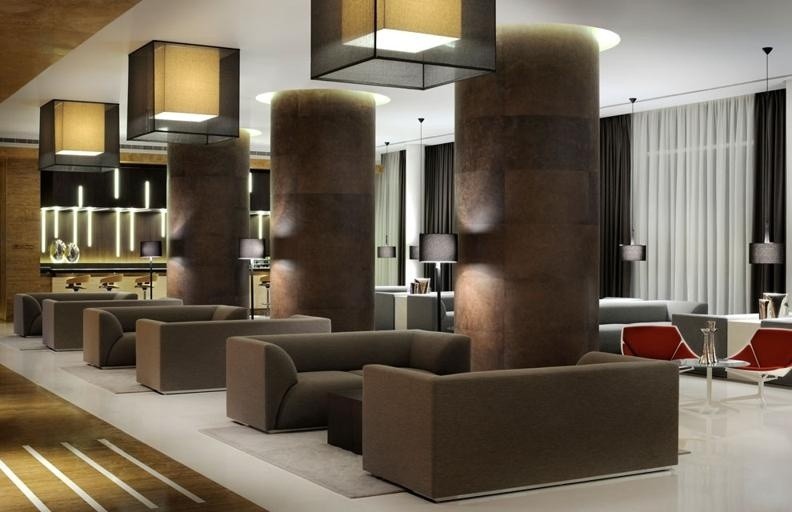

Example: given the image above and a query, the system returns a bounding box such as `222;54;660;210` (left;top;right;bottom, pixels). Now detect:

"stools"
65;273;159;300
258;275;270;317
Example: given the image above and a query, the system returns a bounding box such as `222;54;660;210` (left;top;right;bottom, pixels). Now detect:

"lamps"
377;118;425;260
38;39;241;174
310;0;497;91
618;98;647;262
140;240;162;299
418;233;458;331
748;46;785;266
237;238;266;319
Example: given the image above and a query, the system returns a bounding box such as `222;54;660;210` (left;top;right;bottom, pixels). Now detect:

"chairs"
619;325;792;421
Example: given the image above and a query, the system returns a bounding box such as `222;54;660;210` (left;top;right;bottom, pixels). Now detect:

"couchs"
374;285;455;332
598;296;792;375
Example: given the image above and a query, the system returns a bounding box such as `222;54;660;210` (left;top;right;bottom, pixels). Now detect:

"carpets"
197;425;692;500
